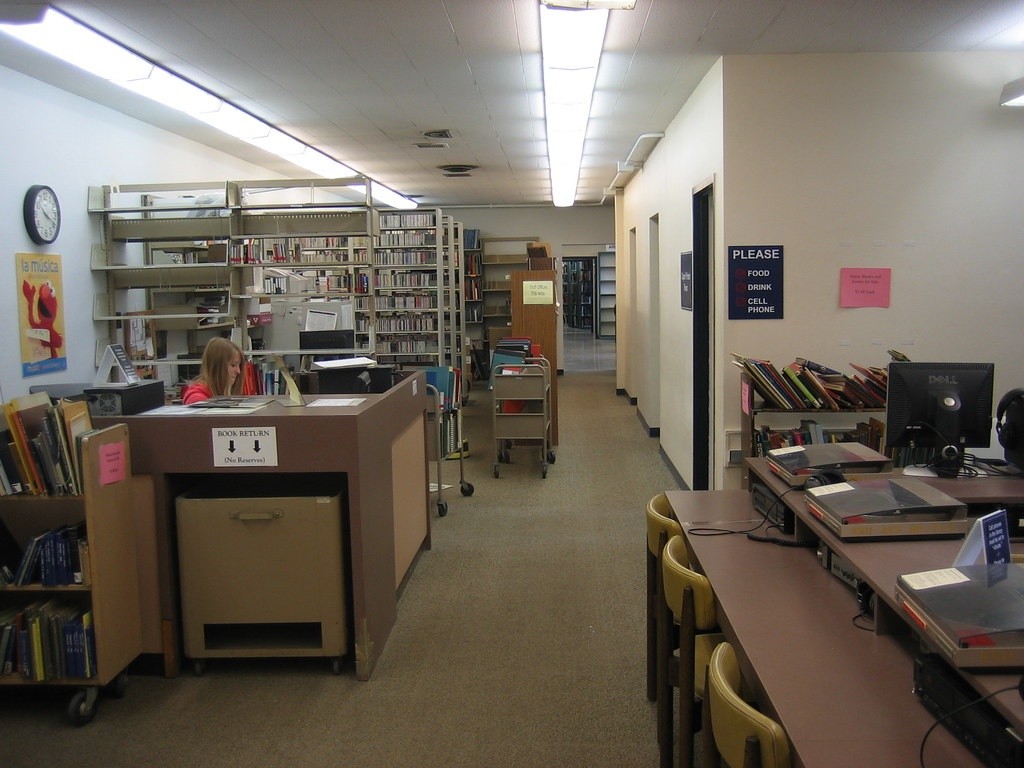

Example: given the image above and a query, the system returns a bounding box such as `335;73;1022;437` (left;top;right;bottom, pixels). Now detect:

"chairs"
657;534;756;768
702;642;792;768
644;493;695;745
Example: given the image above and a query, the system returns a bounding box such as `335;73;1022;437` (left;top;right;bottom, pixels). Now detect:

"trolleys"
427;384;475;517
490;357;556;479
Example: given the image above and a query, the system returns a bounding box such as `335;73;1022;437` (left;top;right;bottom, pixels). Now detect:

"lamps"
0;1;419;210
998;76;1024;108
537;0;609;208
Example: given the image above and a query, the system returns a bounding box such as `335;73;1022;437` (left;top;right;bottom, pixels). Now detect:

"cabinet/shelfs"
510;269;560;448
491;357;557;481
478;236;539;318
201;206;446;368
425;384;475;518
739;372;886;490
562;251;616;340
0;422;144;728
463;248;484;324
314;221;470;409
291;214;458;369
87;178;379;382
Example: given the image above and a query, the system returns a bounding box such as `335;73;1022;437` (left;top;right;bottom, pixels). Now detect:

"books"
0;520;90;587
0;392;103;496
753;417;969;539
487;337;532;389
167;252;199;265
732;348;913;411
564;260;593;328
243;357;294;395
187;291;225;325
0;597;96;681
894;561;1024;668
230;209;485;369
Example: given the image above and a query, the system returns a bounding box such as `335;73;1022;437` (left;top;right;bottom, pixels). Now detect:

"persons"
182;336;246;406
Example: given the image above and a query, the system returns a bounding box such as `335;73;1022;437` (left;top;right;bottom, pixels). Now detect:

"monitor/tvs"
885;361;994;478
298;329;356;375
29;384;92;404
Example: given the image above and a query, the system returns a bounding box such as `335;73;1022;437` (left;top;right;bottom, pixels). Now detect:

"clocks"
23;183;62;247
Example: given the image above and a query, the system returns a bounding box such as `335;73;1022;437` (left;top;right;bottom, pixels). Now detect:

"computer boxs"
314;366;395;393
83;378;166;418
1004;394;1024;470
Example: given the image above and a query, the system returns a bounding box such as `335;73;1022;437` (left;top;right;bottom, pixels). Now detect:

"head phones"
801;469;849;491
996;388;1024;450
